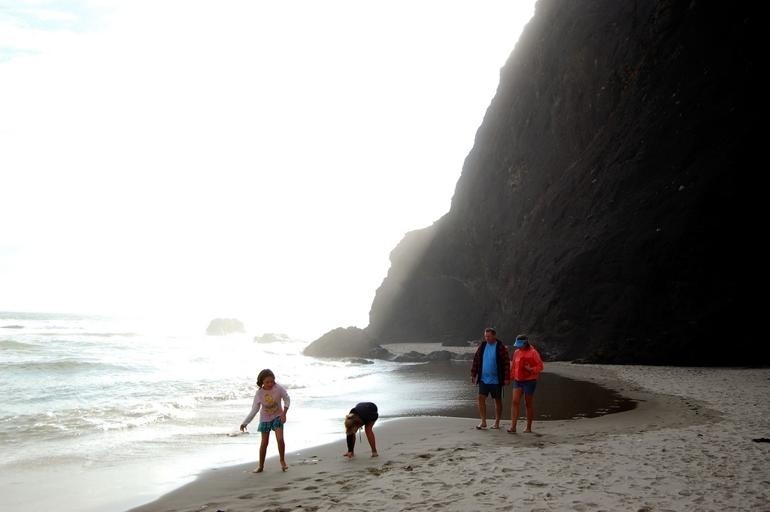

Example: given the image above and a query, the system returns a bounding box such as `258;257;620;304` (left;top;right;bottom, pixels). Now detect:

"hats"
513;338;528;349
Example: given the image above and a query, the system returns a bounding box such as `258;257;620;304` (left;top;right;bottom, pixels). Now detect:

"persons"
343;402;378;458
240;368;289;473
507;334;543;433
470;327;510;429
